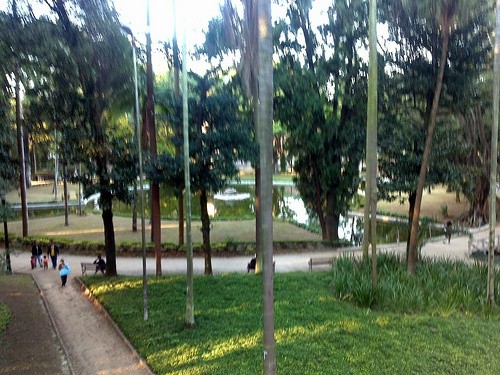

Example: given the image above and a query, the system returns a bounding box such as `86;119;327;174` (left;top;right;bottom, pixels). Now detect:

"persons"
443;222;452;244
94;255;105;273
47;240;59;268
31;257;35;269
58;259;69;286
31;245;37;267
248;254;257;273
37;245;43;267
42;256;48;269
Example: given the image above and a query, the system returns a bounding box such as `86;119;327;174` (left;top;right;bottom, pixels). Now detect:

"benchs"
248;261;275;273
308;256;336;270
81;263;104;275
